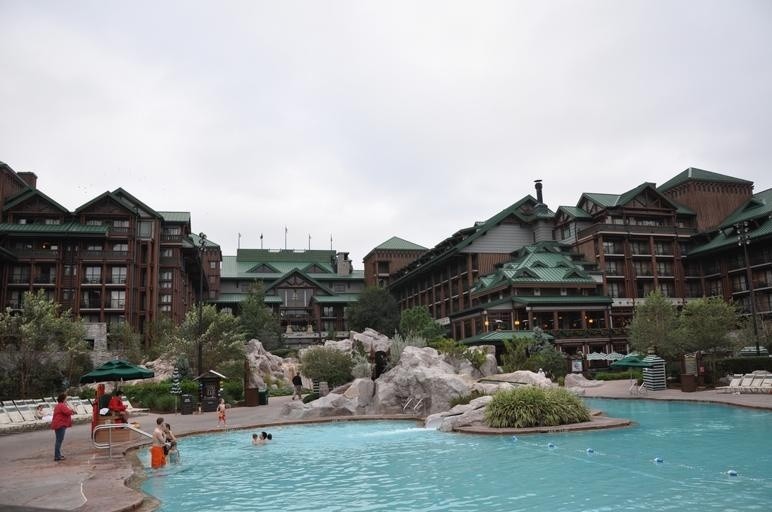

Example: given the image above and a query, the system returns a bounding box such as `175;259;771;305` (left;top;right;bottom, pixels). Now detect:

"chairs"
98;393;125;426
714;372;771;395
1;395;98;435
121;395;150;418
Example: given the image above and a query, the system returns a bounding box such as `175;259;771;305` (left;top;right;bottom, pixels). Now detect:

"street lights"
198;232;207;415
735;220;761;355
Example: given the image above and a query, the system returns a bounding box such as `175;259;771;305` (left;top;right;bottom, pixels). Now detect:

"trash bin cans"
679;374;698;392
181;394;193;415
259;389;270;405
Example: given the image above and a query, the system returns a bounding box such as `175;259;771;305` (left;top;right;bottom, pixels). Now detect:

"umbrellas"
170;367;183;415
79;359;155;390
586;350;652;385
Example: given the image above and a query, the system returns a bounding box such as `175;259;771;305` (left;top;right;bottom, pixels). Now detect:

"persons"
51;394;72;460
151;418;180;468
36;405;48;420
251;431;272;445
107;390;129;423
291;372;303;400
216;398;228;427
